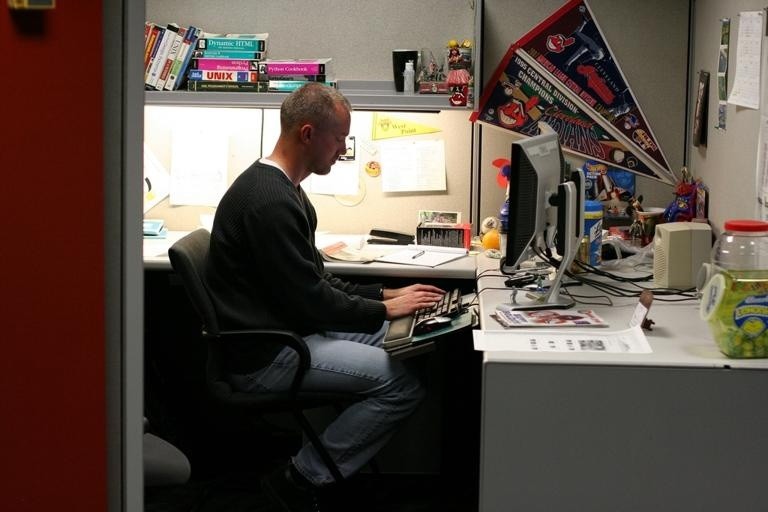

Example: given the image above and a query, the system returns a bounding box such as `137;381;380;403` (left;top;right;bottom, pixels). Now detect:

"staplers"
367;230;416;245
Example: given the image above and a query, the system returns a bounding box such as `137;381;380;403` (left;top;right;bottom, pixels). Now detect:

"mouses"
414;314;451;335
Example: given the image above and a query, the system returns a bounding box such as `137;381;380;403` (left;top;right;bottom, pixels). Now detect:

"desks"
477;237;767;511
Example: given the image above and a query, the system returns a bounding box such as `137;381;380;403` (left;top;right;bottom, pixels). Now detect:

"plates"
636;207;664;221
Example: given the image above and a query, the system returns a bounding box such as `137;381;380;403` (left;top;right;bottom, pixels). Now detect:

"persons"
201;86;447;512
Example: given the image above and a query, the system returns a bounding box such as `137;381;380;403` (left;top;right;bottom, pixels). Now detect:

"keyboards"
414;287;460;325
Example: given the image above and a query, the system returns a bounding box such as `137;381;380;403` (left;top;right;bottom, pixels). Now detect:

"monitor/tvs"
506;133;585;308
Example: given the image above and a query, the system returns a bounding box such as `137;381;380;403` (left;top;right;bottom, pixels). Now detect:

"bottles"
403;62;415;95
696;220;768;359
585;200;604;266
467;82;474;109
500;206;509;258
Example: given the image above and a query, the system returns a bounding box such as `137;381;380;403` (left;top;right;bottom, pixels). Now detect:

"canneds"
572;235;591;272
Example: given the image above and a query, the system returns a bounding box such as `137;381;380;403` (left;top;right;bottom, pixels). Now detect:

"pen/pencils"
412;250;426;261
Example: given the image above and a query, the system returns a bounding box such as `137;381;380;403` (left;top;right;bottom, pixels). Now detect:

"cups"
392;49;418;92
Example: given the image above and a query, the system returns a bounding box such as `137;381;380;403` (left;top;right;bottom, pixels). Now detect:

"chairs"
167;228;381;512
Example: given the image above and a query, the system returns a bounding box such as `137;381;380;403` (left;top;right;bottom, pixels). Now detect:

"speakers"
652;220;714;293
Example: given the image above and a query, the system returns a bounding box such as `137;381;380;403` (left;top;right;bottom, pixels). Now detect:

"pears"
481;219;501;250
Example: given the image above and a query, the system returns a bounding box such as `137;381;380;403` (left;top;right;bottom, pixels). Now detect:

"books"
143;17;341;93
